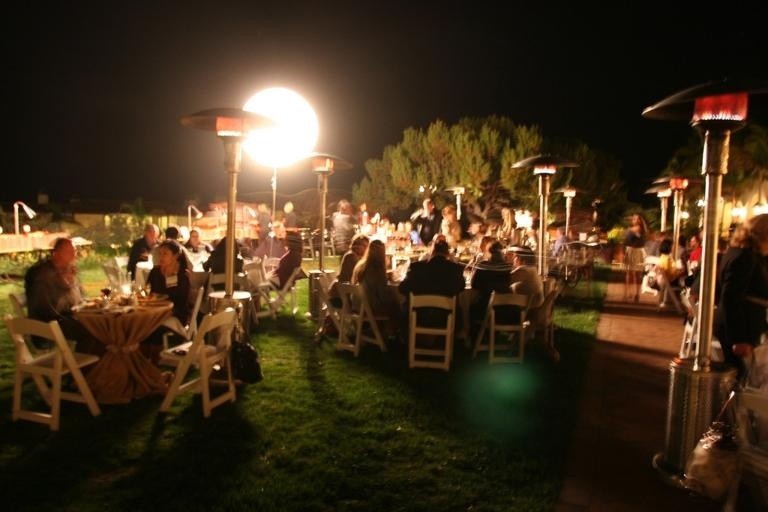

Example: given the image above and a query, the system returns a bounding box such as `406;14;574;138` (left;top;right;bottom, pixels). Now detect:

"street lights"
640;171;703;268
443;182;472;226
639;78;763;477
512;151;589;280
298;146;350;270
176;106;285;295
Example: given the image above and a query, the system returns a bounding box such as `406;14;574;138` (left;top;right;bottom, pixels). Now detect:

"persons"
185;230;206;252
500;208;514;238
716;238;728;264
165;226;181;243
253;203;274;255
329;235;369;310
332;199;359;225
713;212;768;375
689;235;703;264
398;241;466;346
138;240;190;368
471;242;519;342
25;237;106;396
267;230;304;291
440;204;463;242
621;213;647;305
201;236;246;291
505;247;545;342
418;232;458;264
524;227;539;251
408;198;442;242
350;239;400;345
659;238;682;288
282;201;297;230
678;235;689;271
331;202;355;252
127;224;161;283
552;227;569;257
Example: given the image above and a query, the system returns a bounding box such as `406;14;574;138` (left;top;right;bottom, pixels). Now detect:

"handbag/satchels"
233;308;263;382
683;391;740;500
